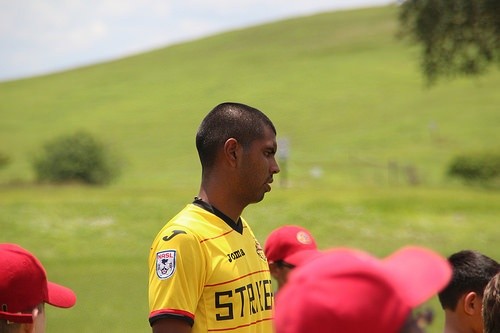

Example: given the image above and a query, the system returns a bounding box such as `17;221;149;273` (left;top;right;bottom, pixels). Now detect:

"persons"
437;250;500;333
148;101;281;333
264;225;324;291
481;271;500;333
273;245;453;333
0;242;76;333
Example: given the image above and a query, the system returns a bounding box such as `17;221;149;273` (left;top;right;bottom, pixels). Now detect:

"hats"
262;224;320;268
273;246;453;332
0;243;76;323
